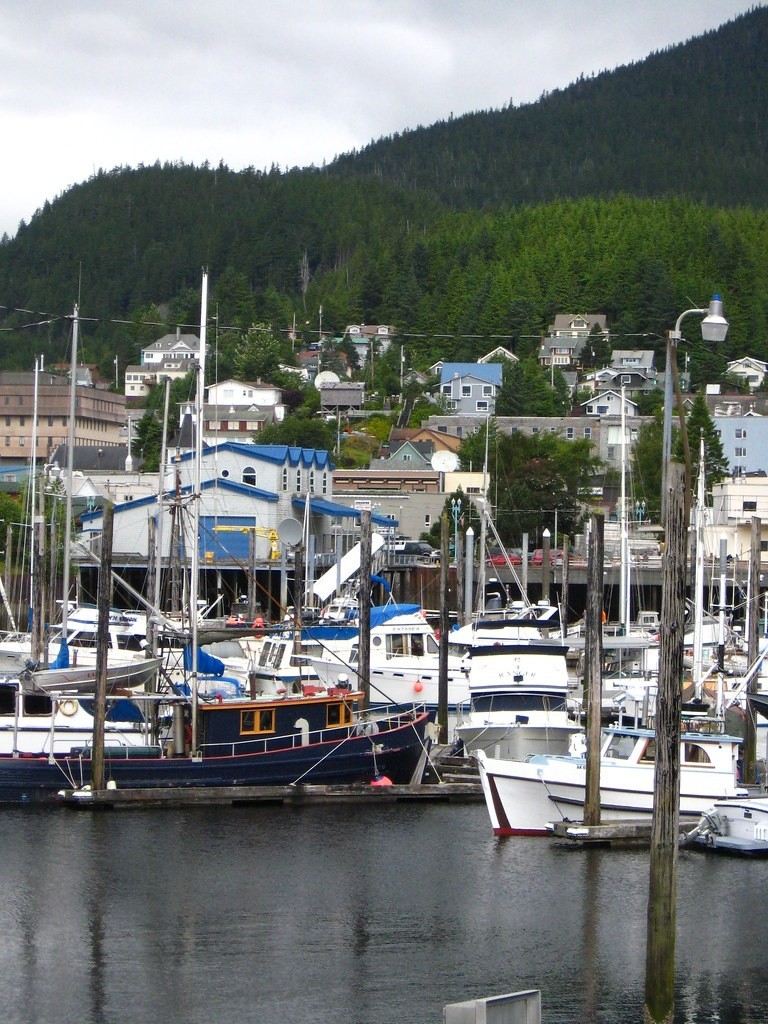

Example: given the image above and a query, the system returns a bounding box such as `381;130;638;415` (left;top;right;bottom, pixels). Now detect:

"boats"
0;259;768;861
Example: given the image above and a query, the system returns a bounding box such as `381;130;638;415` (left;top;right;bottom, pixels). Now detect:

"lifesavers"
252;624;266;638
60;699;79;716
